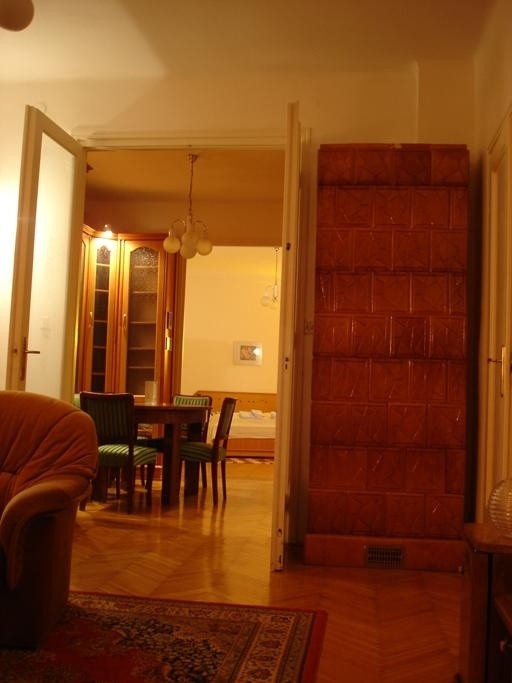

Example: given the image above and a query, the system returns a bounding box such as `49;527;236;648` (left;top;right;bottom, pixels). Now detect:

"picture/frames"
233;340;262;364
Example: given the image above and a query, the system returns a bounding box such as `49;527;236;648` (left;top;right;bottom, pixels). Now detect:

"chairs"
0;389;99;647
72;390;235;513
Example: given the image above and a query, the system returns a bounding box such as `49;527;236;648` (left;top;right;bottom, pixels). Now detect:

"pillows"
239;407;276;420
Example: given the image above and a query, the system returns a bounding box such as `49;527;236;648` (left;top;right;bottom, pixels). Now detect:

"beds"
195;390;277;456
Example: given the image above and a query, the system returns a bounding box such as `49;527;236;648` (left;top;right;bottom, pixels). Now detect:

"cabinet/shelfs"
75;223;173;481
458;519;512;683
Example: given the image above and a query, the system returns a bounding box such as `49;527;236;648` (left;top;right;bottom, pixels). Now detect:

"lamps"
273;246;280;300
101;224;112;232
163;153;212;258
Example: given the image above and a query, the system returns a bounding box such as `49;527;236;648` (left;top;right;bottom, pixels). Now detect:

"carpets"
1;591;327;682
225;458;275;464
